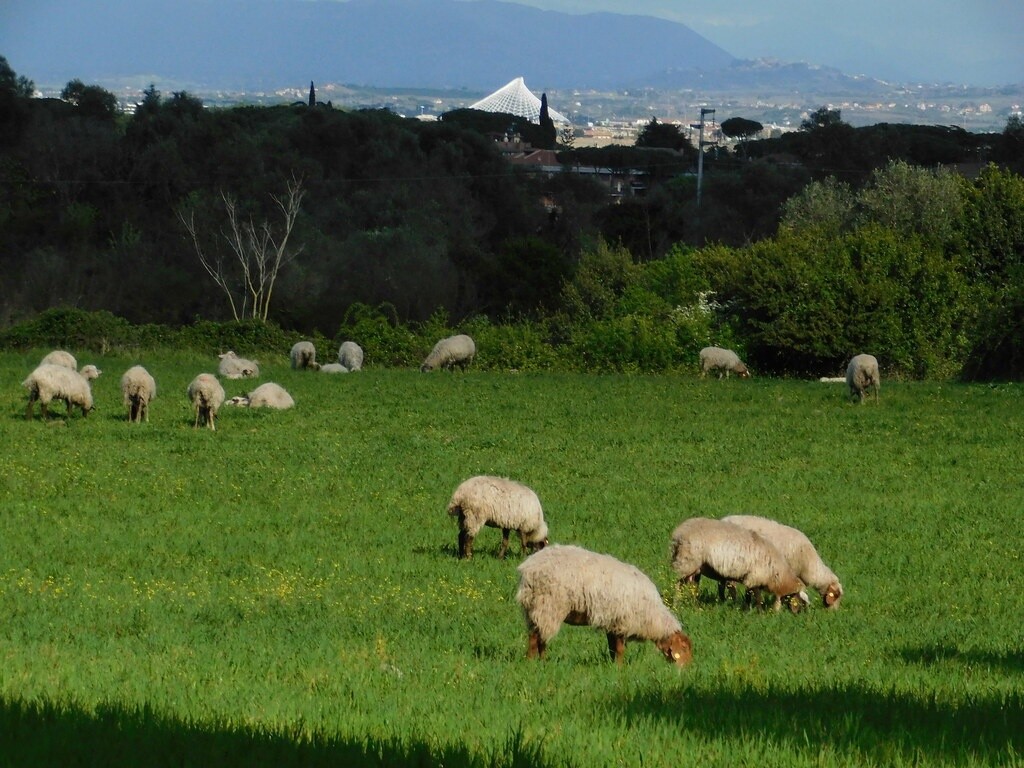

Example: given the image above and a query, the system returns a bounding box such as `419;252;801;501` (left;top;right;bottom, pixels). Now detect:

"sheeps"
289;340;364;374
513;541;694;675
669;513;845;618
225;381;297;412
217;350;260;379
699;346;751;381
446;474;549;561
418;334;476;375
21;348;104;424
846;353;881;406
120;364;157;425
188;373;226;432
